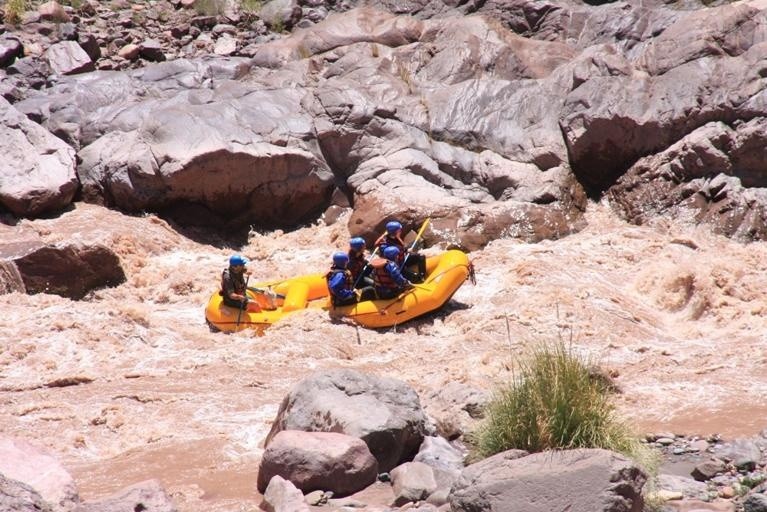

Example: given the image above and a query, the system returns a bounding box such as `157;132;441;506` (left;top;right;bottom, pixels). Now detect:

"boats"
204;250;479;332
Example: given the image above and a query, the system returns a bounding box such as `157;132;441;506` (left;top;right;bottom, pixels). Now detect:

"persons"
219;255;255;309
328;222;426;304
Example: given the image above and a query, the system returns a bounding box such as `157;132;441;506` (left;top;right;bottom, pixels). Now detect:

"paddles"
409;284;432;292
353;232;387;290
401;218;429;270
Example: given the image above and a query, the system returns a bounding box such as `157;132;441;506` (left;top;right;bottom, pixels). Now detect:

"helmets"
230;255;247;265
386;221;402;234
383;246;400;261
332;252;349;268
350;236;365;251
